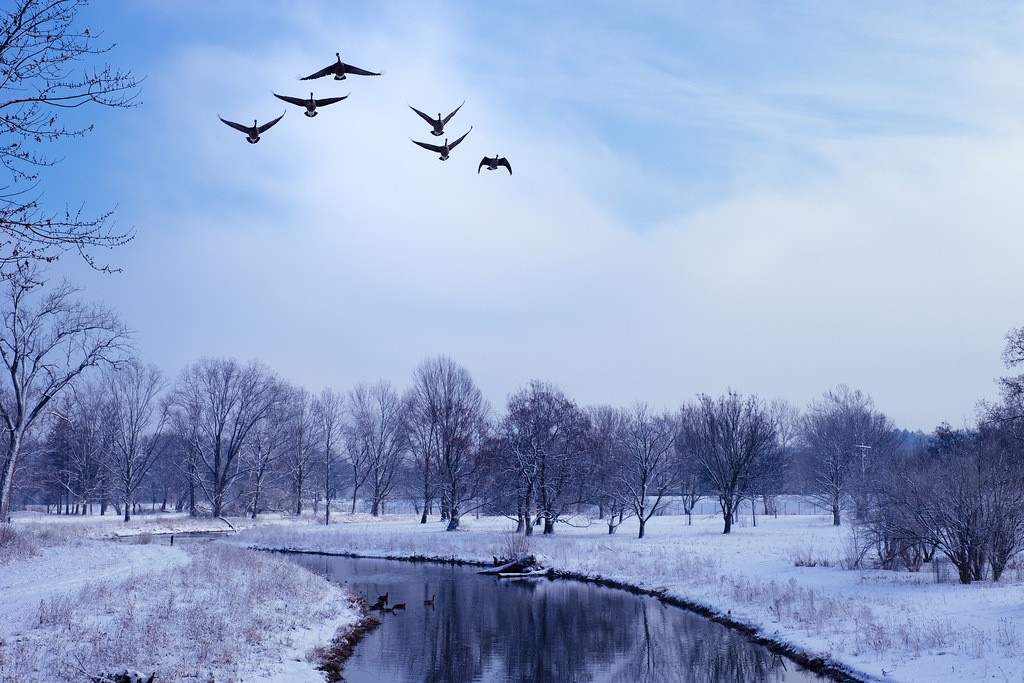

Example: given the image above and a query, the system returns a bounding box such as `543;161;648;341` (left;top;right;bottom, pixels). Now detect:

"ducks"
370;591;406;612
424;594;436;603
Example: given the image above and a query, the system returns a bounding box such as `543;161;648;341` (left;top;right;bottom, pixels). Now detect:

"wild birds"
300;52;381;82
410;125;474;161
270;91;351;118
408;100;466;136
216;108;287;145
477;154;511;175
